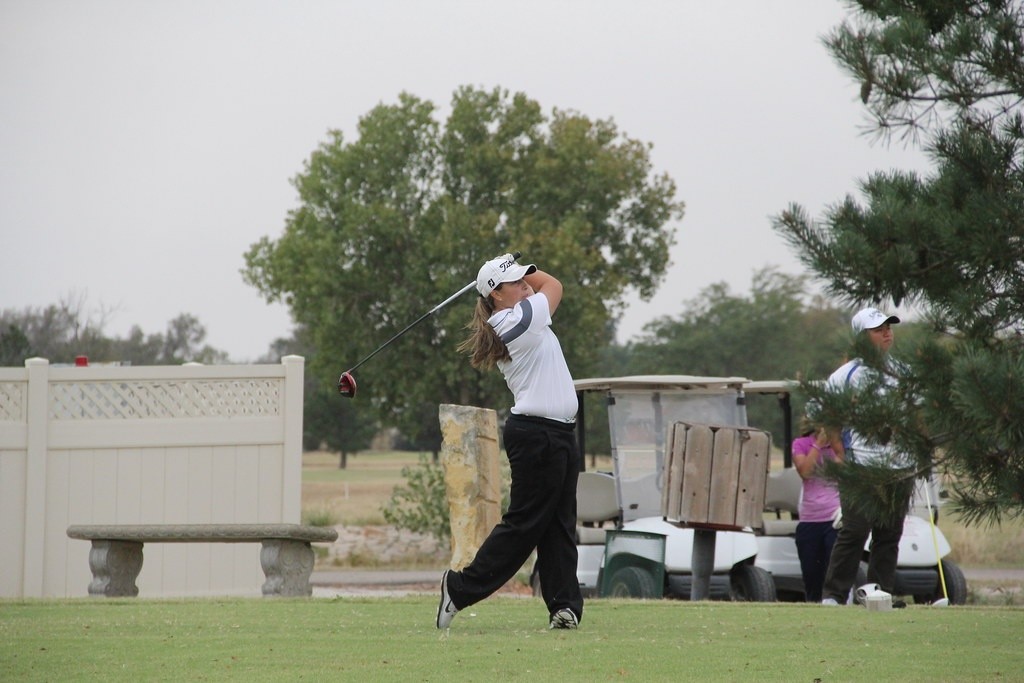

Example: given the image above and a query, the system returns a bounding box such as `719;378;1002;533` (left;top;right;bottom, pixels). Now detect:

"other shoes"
823;599;837;605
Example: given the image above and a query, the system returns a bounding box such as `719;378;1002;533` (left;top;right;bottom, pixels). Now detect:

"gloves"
500;252;514;262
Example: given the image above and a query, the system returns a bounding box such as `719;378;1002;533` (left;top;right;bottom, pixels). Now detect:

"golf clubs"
337;250;523;400
922;475;951;607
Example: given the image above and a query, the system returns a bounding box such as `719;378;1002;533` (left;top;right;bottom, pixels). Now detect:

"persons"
791;398;842;601
437;253;584;628
822;308;915;604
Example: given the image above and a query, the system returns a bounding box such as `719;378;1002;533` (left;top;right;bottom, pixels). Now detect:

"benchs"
66;523;338;596
762;467;801;535
576;472;662;544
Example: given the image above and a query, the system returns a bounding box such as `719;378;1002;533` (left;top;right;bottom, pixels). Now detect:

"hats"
804;397;825;416
852;307;899;335
477;258;538;297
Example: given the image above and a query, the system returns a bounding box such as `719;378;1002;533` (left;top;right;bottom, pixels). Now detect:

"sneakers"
437;569;460;630
550;609;579;629
